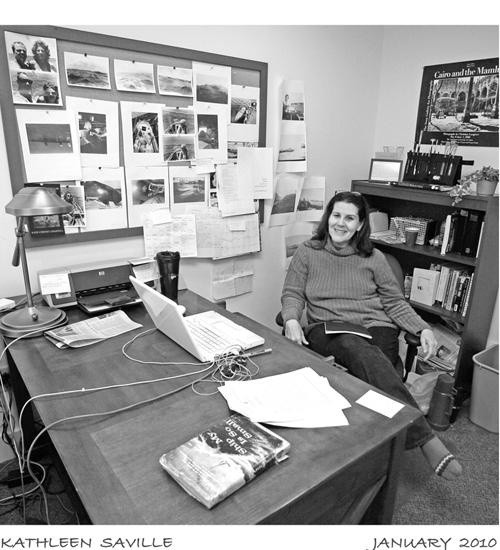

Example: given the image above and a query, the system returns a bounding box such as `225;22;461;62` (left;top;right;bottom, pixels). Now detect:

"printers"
71;263;143;313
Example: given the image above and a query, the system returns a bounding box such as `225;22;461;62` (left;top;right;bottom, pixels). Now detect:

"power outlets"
0;366;13;393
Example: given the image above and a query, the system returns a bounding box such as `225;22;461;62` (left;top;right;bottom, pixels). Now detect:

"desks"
0;290;424;525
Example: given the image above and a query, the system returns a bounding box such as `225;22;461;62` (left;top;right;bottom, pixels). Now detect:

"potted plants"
449;167;499;205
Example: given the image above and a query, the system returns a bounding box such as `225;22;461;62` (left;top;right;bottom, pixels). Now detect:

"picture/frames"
368;159;402;184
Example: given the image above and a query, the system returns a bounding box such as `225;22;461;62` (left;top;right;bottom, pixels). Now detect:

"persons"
35;80;58;104
61;192;77;221
12;40;30;70
279;190;465;483
13;71;33;104
281;93;303;120
30;38;56;73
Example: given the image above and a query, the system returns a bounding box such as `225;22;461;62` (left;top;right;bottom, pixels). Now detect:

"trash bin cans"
469;344;500;434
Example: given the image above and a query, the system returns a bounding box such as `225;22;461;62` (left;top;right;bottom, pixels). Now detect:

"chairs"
277;254;421;383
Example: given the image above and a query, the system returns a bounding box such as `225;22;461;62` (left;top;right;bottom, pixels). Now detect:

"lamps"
0;187;74;339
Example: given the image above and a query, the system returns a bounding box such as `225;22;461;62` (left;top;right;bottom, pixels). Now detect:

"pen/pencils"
242;348;272;358
406;130;457;177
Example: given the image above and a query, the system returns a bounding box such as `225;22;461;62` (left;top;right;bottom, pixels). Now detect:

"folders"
451;208;486;257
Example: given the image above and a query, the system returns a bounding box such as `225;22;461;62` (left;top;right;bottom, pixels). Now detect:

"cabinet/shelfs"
350;179;499;405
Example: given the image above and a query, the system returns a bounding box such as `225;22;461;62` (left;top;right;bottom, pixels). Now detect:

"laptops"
128;275;265;362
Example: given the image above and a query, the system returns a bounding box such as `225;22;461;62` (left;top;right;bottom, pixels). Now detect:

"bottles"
426;373;457;431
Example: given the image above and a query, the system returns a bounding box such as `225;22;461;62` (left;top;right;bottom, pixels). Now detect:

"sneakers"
420;436;464;482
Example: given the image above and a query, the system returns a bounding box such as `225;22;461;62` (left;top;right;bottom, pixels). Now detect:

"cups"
405;227;419;248
154;250;181;304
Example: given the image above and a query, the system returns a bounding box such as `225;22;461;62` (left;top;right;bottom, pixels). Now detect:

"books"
440;213;456;256
400;259;475;317
158;413;293;512
463;211;486;258
452;209;470;253
322;320;373;341
